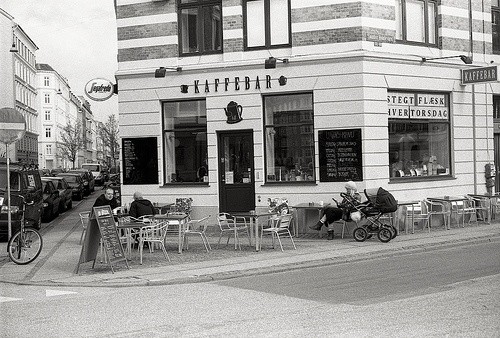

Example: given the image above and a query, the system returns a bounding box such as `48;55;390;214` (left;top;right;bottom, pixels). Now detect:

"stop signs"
0;107;27;145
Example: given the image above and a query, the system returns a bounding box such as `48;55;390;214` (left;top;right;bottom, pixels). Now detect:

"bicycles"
7;194;43;265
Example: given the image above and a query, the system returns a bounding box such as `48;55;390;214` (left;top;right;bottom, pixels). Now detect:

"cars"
40;176;72;213
37;163;120;201
41;179;64;223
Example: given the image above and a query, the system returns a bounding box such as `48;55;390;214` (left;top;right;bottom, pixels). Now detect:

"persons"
128;191;157;248
88;187;119;218
308;181;361;240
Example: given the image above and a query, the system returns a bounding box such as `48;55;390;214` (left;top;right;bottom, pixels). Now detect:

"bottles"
117;203;131;214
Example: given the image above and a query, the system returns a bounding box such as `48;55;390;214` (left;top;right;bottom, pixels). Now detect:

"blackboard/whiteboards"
122;137;159;185
78;205;127;266
318;128;363;182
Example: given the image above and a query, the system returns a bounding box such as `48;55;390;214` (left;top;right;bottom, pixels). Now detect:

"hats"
345;181;357;192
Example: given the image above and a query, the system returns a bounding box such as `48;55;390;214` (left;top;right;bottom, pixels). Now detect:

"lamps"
265;57;289;69
155;67;182;78
423;55;472;64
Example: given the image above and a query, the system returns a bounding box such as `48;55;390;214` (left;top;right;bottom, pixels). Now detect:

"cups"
309;201;313;206
319;201;323;205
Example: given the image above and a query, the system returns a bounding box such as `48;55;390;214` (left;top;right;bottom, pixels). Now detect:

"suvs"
0;166;43;242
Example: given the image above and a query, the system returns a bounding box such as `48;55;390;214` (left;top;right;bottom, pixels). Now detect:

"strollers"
340;187;397;243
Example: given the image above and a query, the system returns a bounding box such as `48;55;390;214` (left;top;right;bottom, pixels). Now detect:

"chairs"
79;209;212;265
216;206;297;253
334;192;500;240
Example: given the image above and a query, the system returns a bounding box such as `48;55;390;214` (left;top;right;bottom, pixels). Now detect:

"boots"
309;221;323;231
327;231;334;240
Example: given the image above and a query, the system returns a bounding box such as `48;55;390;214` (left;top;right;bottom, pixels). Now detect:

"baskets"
25;205;42;222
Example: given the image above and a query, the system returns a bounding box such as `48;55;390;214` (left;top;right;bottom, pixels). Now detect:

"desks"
292;203;331;238
113;213;129;225
468;194;500;225
427;198;468;230
393;202;419;236
154;214;188;253
117;222;144;260
231;212;275;252
153;203;176;215
